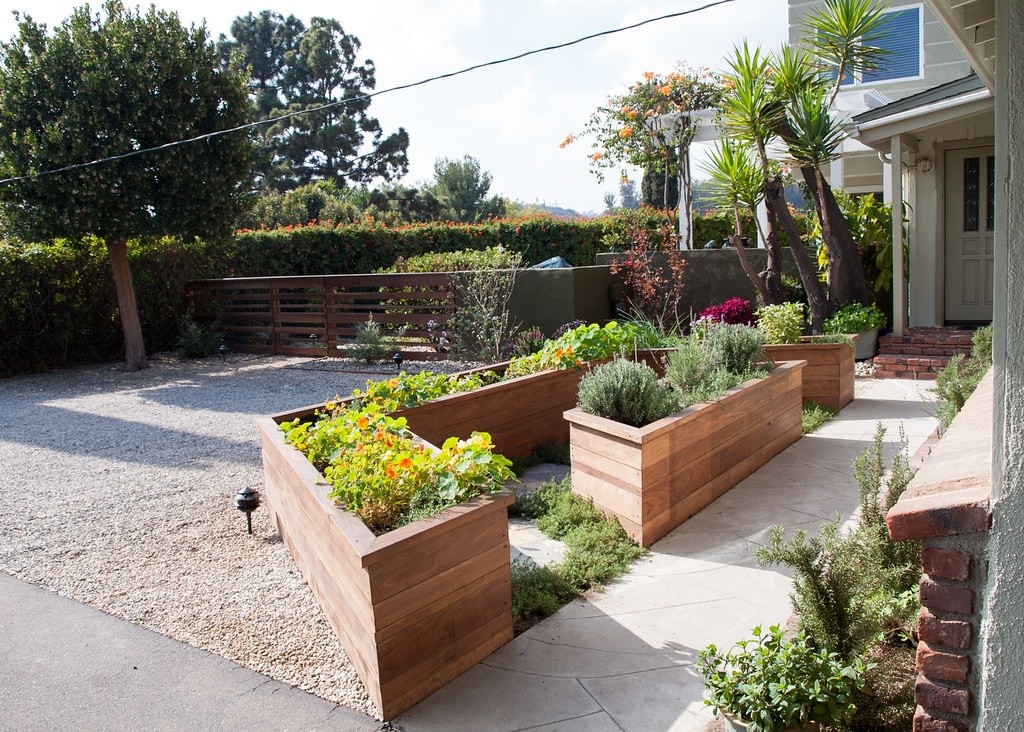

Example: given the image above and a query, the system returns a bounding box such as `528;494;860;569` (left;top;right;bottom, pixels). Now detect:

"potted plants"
821;302;886;359
